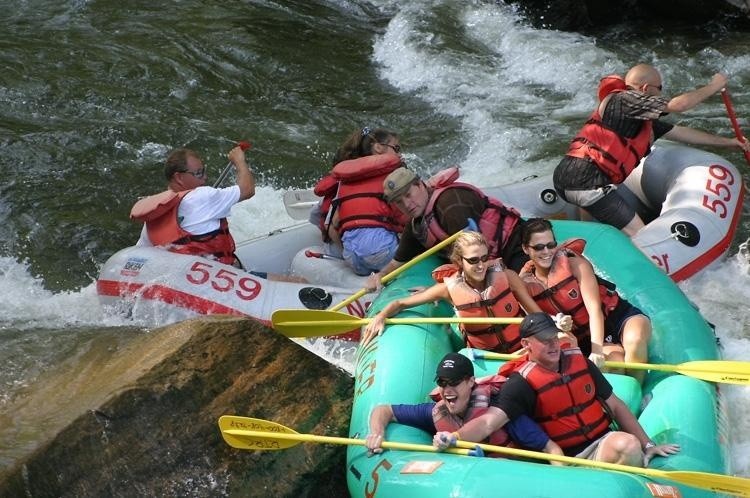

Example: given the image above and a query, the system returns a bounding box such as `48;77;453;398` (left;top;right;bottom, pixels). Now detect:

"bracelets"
452;431;463;442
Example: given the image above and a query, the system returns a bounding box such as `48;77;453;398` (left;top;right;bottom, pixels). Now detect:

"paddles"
473;347;750;385
271;310;559;338
440;434;750;497
219;415;483;457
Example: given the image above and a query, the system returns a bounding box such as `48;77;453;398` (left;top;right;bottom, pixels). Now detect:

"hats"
433;352;474;383
519;312;564;341
383;167;421;205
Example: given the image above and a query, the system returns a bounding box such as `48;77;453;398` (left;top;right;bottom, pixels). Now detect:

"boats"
96;139;742;342
345;220;727;498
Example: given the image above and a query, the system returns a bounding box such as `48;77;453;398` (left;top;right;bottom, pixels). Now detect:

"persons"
128;146;310;284
362;228;575;353
361;165;530;293
364;353;563;466
315;127;408;275
551;61;749;235
516;219;654;386
433;312;682;474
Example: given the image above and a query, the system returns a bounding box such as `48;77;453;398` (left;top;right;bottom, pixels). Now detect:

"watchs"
642;441;656;451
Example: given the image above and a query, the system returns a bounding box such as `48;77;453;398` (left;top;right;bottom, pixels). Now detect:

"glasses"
528;241;557;251
380;144;401;153
436;378;466;387
640;84;662;91
460;254;489;264
175;167;205;178
